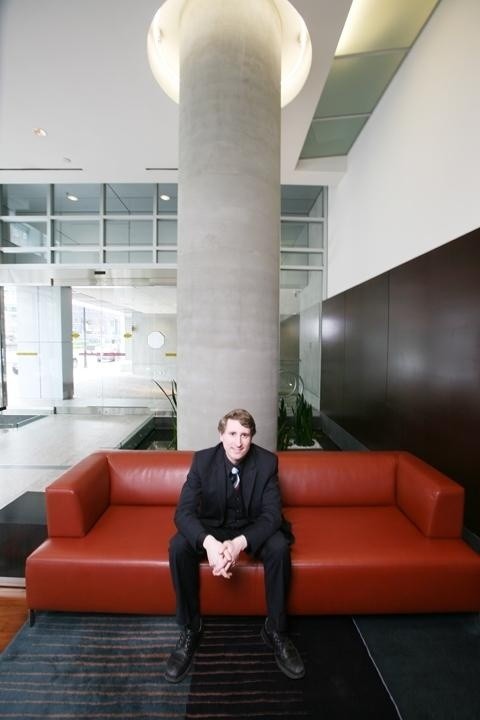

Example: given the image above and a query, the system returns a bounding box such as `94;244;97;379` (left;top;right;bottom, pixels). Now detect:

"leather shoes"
165;618;204;682
260;616;305;680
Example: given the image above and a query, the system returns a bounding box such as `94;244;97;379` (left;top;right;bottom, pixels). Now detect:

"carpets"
1;609;480;719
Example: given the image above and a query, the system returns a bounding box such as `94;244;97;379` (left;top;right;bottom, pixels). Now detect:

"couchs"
23;448;479;629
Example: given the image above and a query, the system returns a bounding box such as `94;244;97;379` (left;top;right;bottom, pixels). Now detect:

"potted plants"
277;392;324;451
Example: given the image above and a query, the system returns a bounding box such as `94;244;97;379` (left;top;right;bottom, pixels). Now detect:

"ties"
230;467;241;493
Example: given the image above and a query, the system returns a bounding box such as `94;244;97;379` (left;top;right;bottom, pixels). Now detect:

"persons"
165;408;306;683
